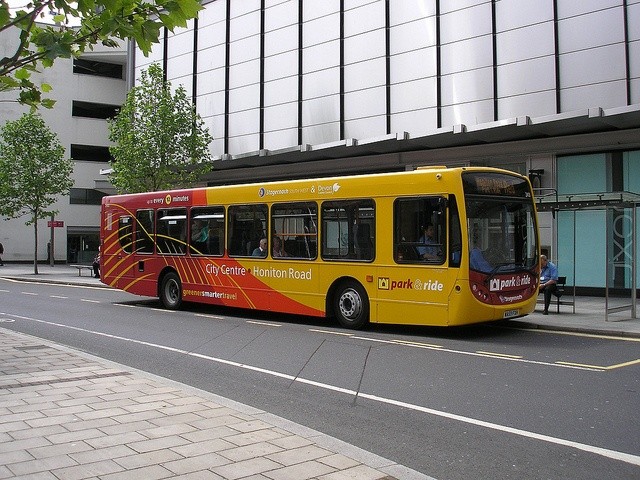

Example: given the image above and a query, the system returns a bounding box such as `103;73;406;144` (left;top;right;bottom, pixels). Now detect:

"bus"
98;165;542;329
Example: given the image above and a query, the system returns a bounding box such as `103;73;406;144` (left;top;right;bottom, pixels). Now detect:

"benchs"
70;264;94;277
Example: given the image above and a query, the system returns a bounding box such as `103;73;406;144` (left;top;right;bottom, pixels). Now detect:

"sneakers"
543;310;548;314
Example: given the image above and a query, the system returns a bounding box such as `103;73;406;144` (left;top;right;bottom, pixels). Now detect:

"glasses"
260;243;267;244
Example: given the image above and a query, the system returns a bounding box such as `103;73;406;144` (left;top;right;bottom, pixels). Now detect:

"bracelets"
544;284;546;286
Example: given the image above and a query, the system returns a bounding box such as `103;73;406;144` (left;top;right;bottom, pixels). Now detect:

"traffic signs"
48;221;64;227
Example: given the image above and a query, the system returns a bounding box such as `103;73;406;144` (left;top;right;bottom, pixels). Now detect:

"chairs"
553;276;566;313
248;241;258;256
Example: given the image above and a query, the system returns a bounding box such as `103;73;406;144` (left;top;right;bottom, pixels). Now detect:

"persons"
538;255;558;316
0;243;4;266
251;238;267;257
191;209;218;242
92;246;100;278
416;222;441;259
46;239;56;264
273;237;288;257
